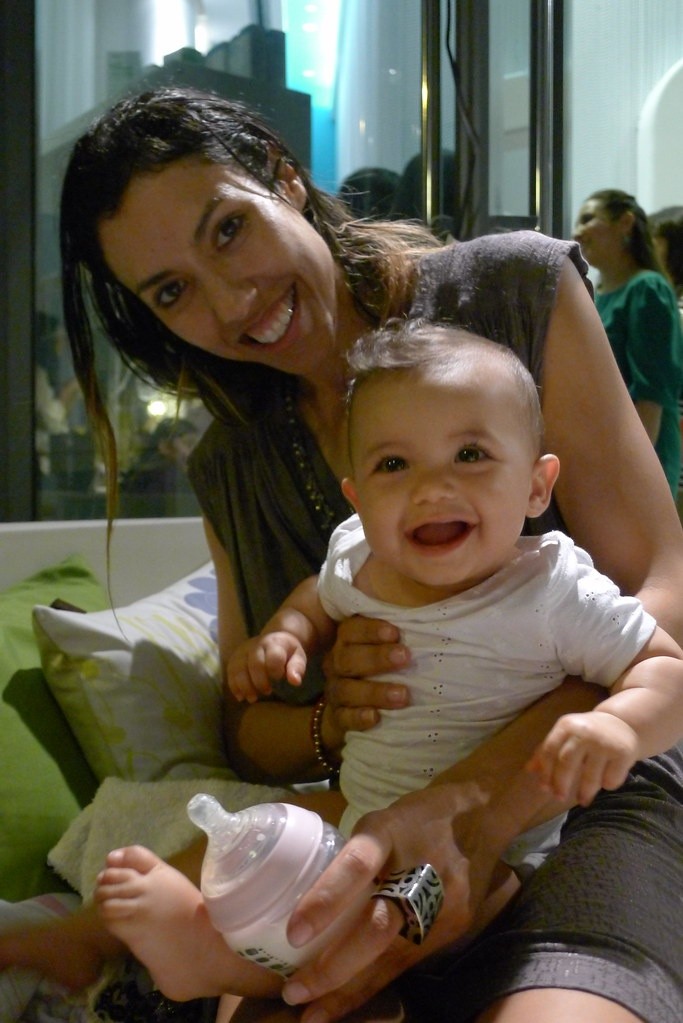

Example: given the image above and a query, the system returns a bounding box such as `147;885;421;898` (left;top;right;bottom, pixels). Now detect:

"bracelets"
309;701;338;772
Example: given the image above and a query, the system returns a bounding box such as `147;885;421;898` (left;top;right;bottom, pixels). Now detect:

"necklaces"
285;383;334;526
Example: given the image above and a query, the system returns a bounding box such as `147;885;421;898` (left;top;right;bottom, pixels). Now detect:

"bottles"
187;794;384;982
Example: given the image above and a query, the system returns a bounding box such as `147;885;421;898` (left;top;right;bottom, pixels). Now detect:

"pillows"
29;556;232;788
0;564;119;902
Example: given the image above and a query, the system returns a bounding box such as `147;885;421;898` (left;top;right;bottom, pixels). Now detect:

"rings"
370;865;445;947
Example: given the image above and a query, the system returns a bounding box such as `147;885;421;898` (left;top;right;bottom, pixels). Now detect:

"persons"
34;313;82;477
0;320;682;1000
573;189;683;507
119;416;197;519
337;148;460;232
59;85;683;1023
649;205;682;305
